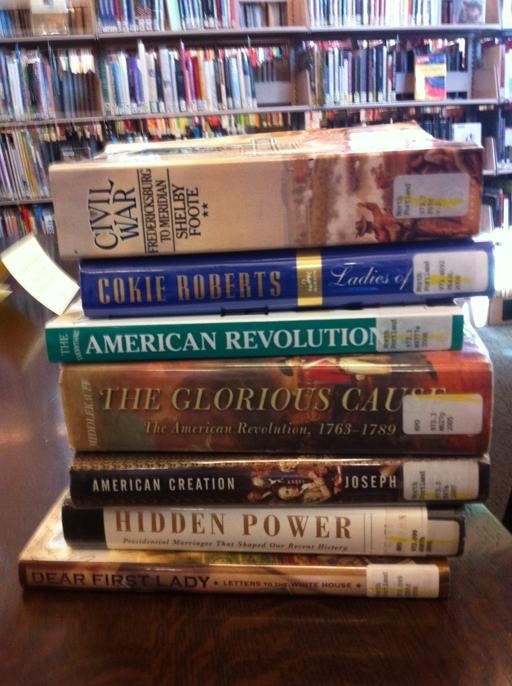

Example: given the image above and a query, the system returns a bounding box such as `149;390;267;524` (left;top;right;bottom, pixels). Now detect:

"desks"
2;227;512;686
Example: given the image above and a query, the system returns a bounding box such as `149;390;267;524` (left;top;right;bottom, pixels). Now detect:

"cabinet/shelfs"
0;22;512;281
